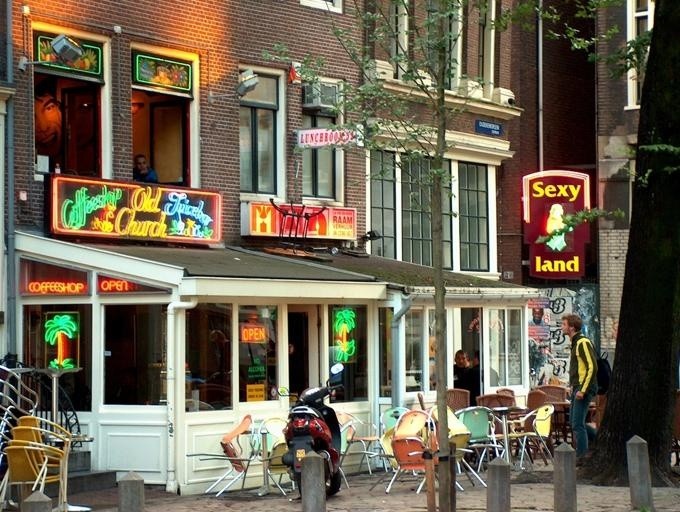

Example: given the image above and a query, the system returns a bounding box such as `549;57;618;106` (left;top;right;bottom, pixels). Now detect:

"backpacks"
574;338;610;394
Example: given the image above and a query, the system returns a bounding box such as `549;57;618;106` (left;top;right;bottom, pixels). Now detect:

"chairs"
0;415;73;511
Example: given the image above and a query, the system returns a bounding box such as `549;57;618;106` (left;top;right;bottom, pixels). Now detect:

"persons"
458;348;499;405
454;349;465;377
528;307;551;349
133;154;159;185
209;329;231;385
34;85;64;157
560;314;600;466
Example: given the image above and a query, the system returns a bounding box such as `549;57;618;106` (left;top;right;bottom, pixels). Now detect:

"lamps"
207;68;259;104
18;34;84;72
357;229;381;248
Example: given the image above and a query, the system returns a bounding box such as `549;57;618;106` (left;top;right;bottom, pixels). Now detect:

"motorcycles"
277;362;346;502
